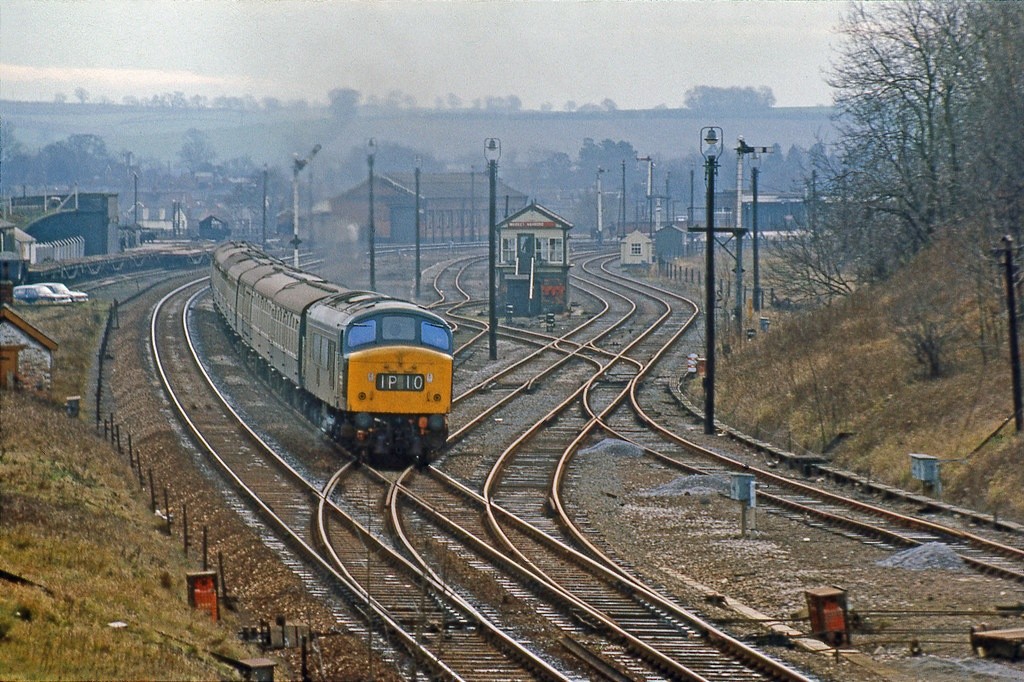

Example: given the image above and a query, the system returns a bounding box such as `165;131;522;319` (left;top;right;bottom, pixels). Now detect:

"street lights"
412;153;422;299
699;125;724;436
483;137;501;361
363;135;379;292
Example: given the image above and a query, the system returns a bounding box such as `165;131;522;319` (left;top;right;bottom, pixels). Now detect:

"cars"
33;283;89;303
13;284;72;304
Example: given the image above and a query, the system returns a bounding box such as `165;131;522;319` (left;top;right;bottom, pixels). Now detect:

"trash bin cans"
185;571;220;622
804;584;850;649
0;344;19;391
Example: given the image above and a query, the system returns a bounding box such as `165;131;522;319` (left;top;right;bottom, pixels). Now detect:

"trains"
209;241;455;471
